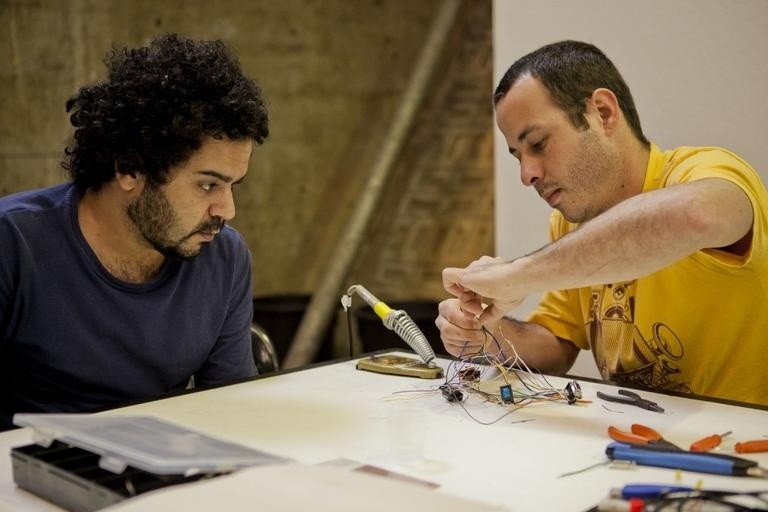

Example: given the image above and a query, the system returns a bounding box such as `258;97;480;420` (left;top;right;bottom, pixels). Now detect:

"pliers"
597;389;664;413
608;424;683;452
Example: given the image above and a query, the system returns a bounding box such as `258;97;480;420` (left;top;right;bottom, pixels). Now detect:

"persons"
434;39;768;411
0;30;270;434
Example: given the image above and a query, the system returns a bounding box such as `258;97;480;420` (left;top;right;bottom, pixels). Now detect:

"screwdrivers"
690;431;732;453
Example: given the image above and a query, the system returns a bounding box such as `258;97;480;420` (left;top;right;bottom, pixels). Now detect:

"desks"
0;347;768;511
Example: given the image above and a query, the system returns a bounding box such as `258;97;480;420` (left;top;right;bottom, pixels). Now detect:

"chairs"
250;324;279;373
